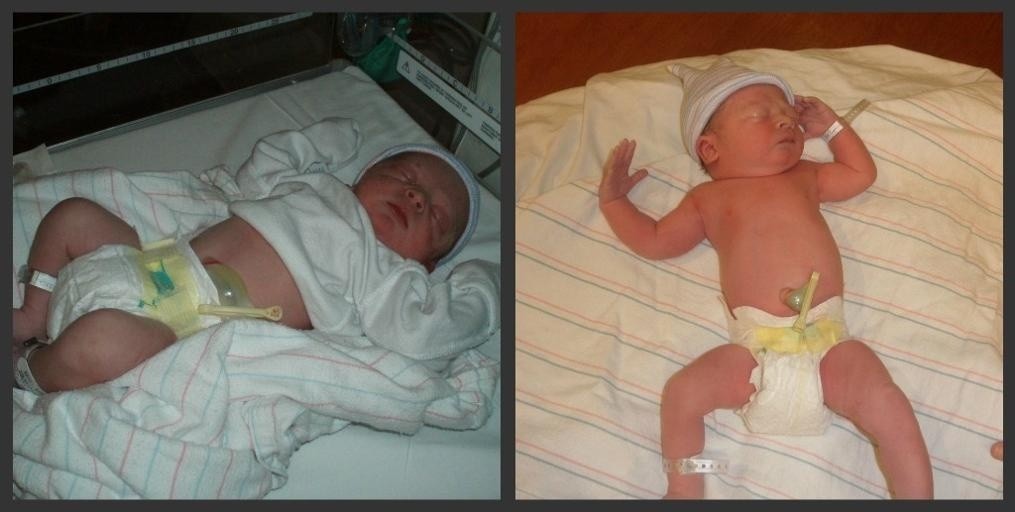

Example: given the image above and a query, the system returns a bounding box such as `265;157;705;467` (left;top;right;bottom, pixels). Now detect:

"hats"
667;56;795;165
352;143;479;269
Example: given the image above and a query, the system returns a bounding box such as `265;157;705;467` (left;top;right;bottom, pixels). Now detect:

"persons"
11;115;501;392
598;55;933;501
